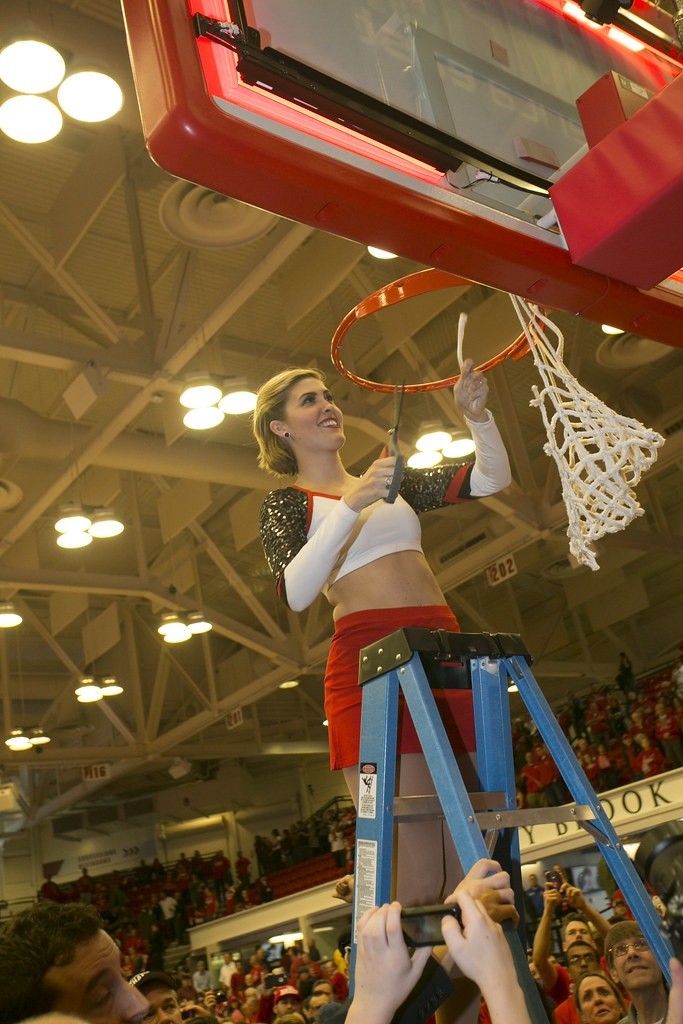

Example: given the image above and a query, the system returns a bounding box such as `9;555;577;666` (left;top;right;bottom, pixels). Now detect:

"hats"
128;970;176;989
614;889;625;898
274;985;304;1005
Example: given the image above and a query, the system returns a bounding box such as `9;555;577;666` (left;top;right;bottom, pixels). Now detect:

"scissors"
358;377;417;505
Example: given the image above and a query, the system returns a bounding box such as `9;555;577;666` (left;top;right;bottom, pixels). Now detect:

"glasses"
569;955;598;965
609;938;650;957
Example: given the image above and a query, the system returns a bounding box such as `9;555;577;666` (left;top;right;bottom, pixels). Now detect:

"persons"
512;653;683;811
131;925;353;1023
252;359;512;1024
0;858;519;1024
481;866;683;1024
345;890;533;1024
42;806;357;980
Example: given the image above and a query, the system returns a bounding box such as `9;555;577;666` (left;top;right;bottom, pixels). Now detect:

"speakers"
168;761;192;779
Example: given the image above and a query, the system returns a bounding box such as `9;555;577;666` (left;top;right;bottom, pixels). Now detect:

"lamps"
29;726;51;745
507;679;519;692
74;675;100;696
185;611;212;635
87;508;124;538
157;611;186;636
0;601;23;628
217;375;258;415
413;419;453;452
100;675;124;696
405;445;444;468
168;760;192;779
440;427;476;459
180;367;222;408
4;728;29;746
54;503;93;533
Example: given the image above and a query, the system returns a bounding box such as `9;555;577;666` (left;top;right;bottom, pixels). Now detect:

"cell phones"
544;870;566;899
396;902;465;948
181;1010;190;1019
264;974;289;990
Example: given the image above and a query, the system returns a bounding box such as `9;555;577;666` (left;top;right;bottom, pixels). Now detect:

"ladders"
349;630;680;1024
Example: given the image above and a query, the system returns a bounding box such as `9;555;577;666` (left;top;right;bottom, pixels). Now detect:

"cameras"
633;821;683;966
212;990;228;1003
